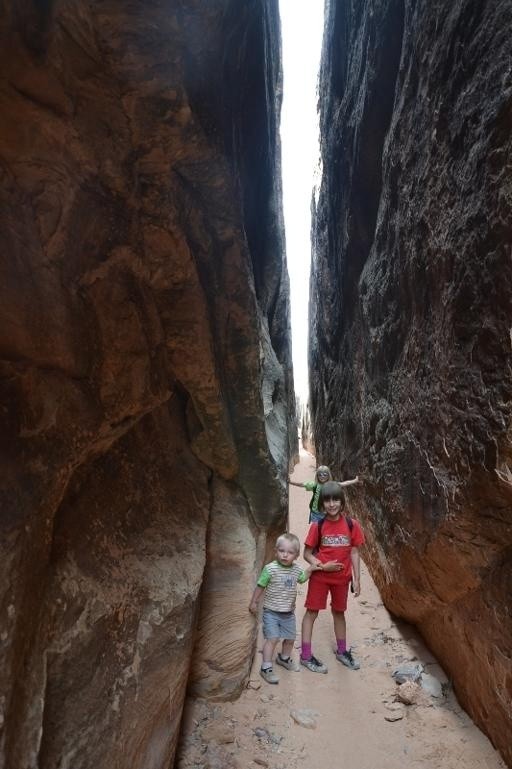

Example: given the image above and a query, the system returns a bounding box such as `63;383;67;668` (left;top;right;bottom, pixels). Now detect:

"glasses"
318;473;328;476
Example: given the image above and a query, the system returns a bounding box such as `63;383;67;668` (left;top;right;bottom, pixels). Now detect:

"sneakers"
336;650;360;669
300;654;327;673
259;665;278;683
276;653;296;670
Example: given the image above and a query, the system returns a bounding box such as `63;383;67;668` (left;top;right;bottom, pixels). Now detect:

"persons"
249;533;323;684
287;464;359;523
300;480;362;674
